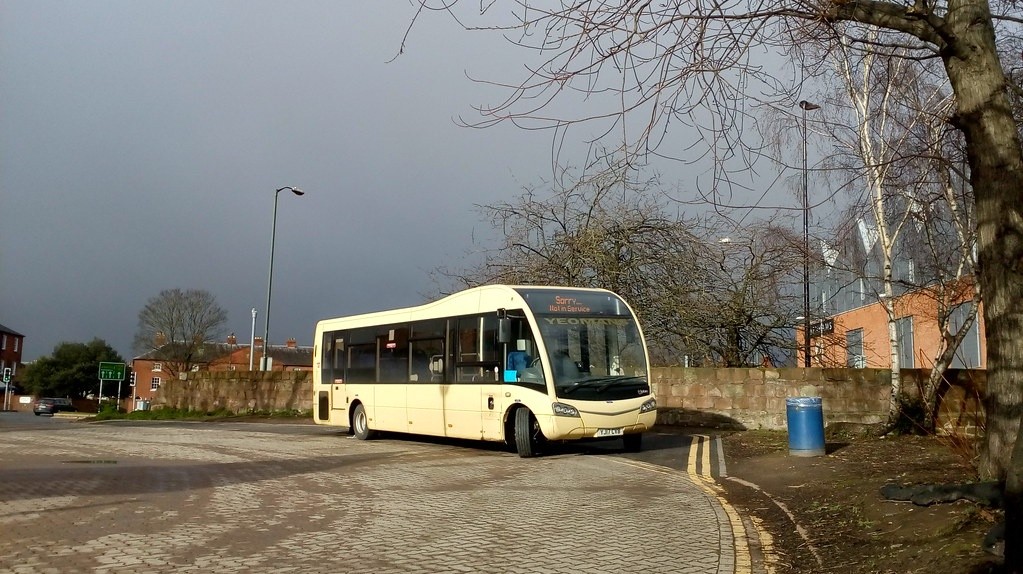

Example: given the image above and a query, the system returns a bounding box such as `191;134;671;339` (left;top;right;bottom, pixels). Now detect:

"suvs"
33;398;78;416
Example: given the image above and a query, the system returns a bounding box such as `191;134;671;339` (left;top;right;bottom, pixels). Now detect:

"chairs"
460;353;480;381
508;352;534;377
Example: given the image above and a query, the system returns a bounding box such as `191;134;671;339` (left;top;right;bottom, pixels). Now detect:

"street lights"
264;185;306;371
717;238;759;367
798;101;820;367
879;294;915;371
796;317;823;367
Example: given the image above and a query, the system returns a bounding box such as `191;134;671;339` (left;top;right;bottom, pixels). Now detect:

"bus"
311;283;658;456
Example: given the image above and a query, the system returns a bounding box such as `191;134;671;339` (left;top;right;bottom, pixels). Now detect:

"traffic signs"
98;361;126;381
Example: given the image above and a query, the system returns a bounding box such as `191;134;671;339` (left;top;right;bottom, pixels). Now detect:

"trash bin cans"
786;396;826;458
136;399;151;410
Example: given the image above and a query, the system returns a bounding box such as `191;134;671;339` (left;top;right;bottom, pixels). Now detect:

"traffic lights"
3;368;10;383
129;371;136;387
